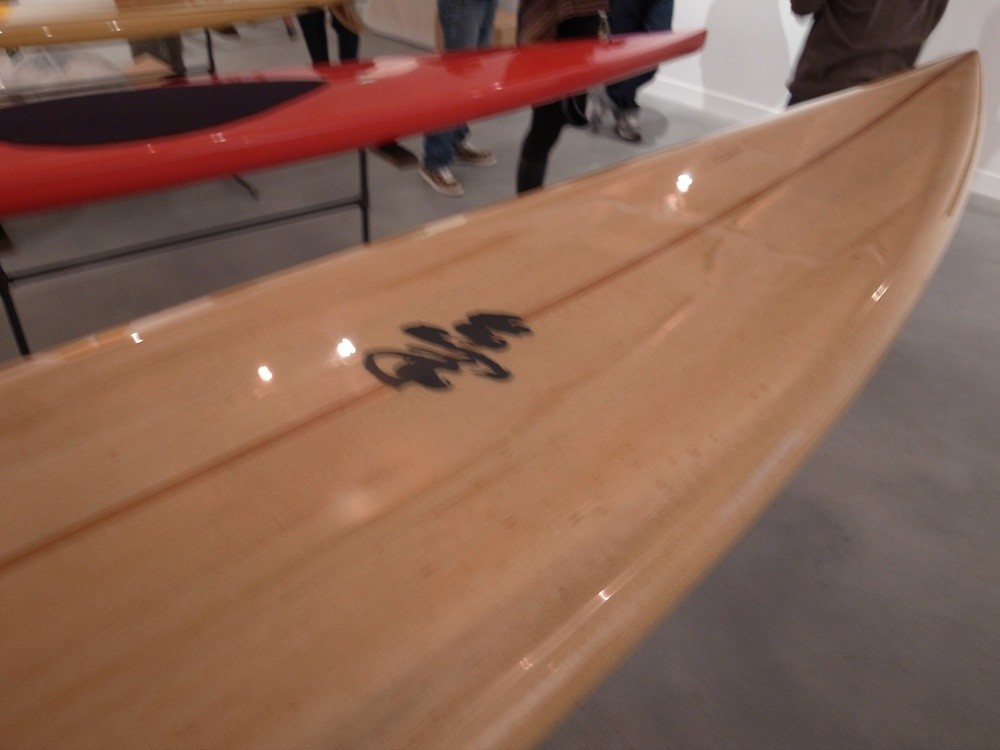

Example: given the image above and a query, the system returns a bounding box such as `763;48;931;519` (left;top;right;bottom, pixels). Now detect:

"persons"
294;0;674;197
787;0;949;106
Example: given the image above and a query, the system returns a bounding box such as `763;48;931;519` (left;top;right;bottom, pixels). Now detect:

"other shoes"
453;138;497;166
425;165;464;196
618;110;641;142
